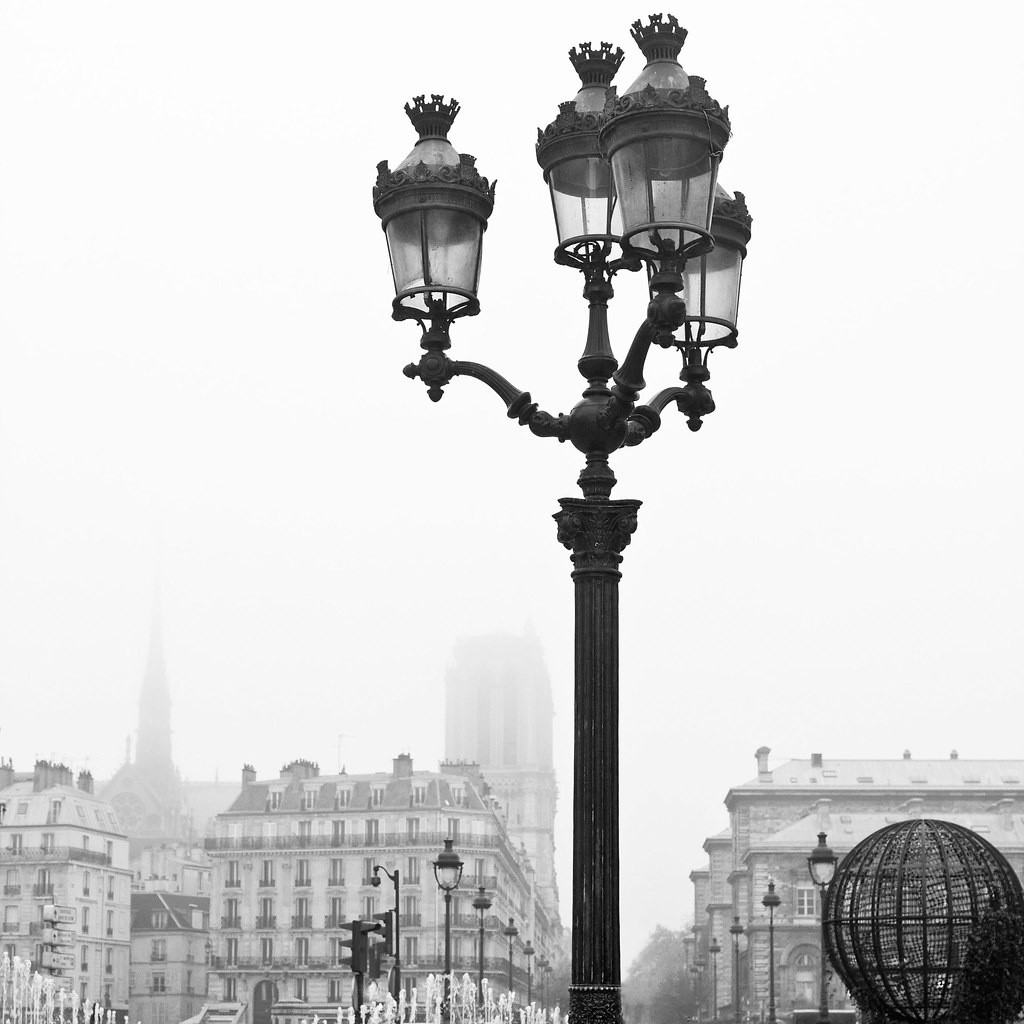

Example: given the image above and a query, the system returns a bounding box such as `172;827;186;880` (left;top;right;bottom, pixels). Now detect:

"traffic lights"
359;920;381;973
373;912;393;955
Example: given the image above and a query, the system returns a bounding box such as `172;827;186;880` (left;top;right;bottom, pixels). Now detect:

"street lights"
433;837;464;1024
709;937;721;1024
522;940;535;1006
689;952;705;1024
503;917;519;1012
471;885;493;1024
807;832;839;1024
729;916;745;1024
538;954;552;1020
762;881;782;1024
373;14;782;1024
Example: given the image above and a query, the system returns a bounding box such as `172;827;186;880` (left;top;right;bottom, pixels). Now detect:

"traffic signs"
42;928;76;946
43;904;77;923
41;973;73;992
42;951;75;968
